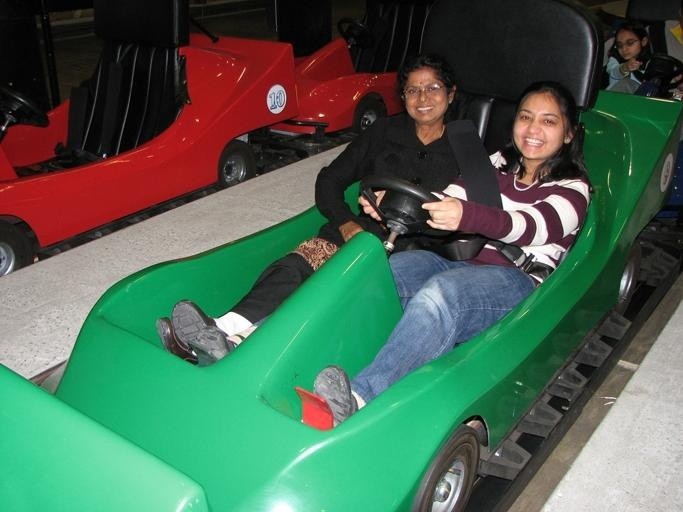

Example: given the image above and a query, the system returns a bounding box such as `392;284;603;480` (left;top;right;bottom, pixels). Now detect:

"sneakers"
313;366;357;426
155;298;235;367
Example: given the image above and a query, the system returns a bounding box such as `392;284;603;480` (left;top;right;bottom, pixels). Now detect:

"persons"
155;48;506;370
600;20;656;94
301;76;592;432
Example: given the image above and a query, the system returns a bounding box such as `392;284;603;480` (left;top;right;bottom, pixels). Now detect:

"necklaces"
511;159;553;192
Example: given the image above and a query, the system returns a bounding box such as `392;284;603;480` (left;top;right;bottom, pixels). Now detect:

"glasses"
614;39;639;49
403;81;446;98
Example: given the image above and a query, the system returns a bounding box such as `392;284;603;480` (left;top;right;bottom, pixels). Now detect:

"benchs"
349;0;436;73
68;0;189;157
420;0;604;155
